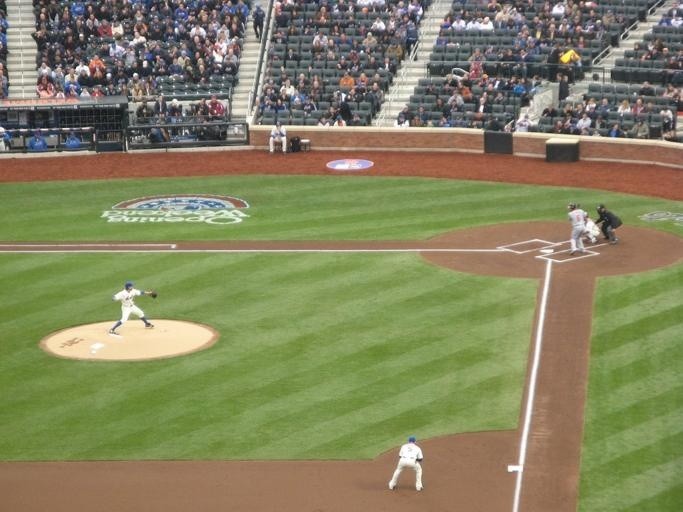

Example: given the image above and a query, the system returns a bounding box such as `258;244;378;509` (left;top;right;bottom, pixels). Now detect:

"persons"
388;436;425;492
595;204;623;245
568;201;585;255
583;212;601;244
108;282;155;336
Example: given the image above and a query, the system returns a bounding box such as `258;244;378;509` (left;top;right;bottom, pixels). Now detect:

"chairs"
0;1;682;152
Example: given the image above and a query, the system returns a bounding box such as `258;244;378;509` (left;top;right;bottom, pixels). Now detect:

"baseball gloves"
150;292;157;298
